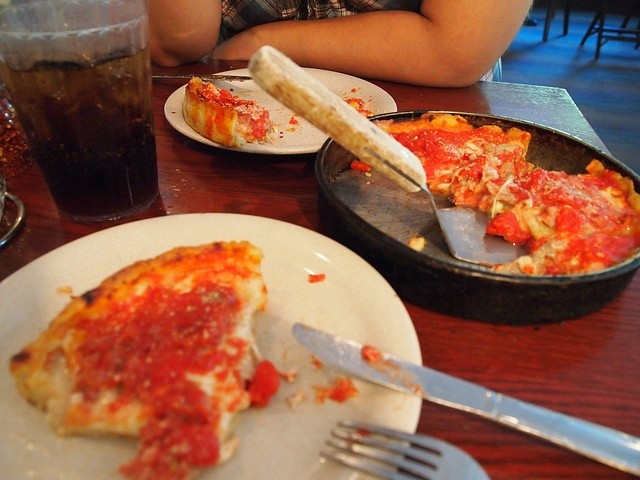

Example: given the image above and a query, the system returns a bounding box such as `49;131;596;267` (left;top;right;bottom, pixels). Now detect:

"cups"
2;2;162;222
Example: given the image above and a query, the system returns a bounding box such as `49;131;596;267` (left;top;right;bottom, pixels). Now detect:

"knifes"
150;74;251;82
291;323;639;476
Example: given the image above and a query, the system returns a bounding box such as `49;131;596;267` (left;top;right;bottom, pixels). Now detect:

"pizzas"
181;76;274;147
9;241;279;479
371;111;636;274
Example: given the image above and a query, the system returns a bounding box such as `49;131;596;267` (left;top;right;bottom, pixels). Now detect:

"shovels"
247;44;534;270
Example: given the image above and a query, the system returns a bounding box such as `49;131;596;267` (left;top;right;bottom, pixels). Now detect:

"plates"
1;211;423;478
162;66;400;155
308;109;639;320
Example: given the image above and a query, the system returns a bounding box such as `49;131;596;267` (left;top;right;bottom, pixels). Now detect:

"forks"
314;413;488;480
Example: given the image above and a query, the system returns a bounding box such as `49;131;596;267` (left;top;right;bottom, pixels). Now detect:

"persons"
146;0;534;87
